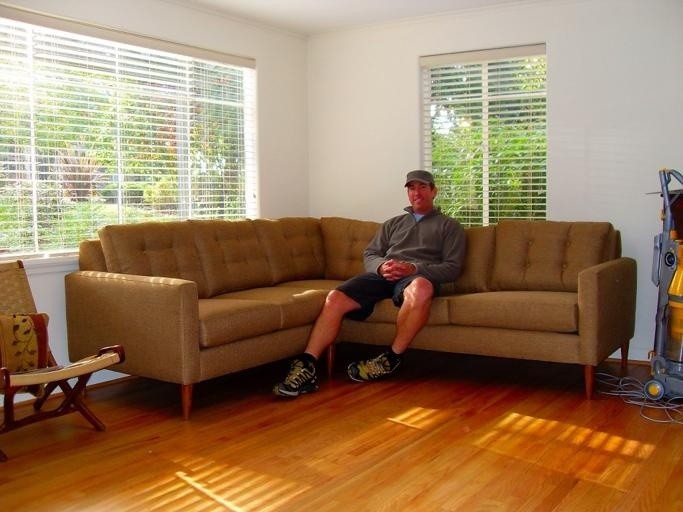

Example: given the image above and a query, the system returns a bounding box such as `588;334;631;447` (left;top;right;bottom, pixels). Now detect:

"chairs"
0;260;126;463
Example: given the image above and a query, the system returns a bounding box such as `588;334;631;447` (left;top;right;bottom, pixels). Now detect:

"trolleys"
644;167;683;403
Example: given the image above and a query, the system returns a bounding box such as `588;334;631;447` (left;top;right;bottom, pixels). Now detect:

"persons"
273;170;466;398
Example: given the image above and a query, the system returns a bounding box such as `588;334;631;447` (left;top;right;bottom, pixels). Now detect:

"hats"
404;170;433;186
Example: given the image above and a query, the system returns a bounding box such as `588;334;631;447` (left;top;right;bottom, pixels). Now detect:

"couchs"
64;215;637;419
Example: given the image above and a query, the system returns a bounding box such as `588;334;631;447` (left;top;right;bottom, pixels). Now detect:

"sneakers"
348;349;402;382
273;357;320;397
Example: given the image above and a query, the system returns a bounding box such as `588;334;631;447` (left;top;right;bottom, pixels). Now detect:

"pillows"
489;219;611;293
251;216;327;285
187;218;275;298
319;217;383;281
0;313;50;373
439;225;496;296
97;219;209;299
